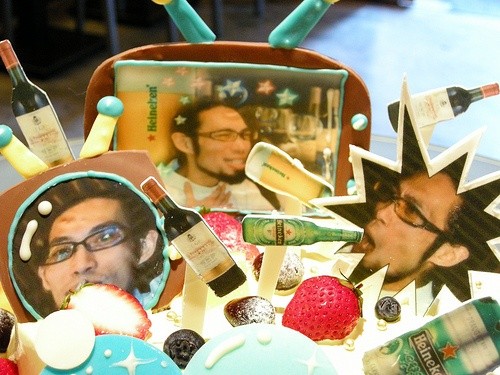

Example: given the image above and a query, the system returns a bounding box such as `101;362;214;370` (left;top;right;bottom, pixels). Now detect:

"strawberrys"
198;207;243;249
58;282;153;341
281;268;365;343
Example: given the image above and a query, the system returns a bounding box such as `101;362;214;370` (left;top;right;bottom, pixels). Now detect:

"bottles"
141;177;246;298
388;82;500;134
241;214;361;245
0;40;76;169
363;296;500;375
422;328;500;374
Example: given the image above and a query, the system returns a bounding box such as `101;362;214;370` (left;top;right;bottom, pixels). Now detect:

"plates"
34;310;95;370
183;325;336;374
41;335;181;375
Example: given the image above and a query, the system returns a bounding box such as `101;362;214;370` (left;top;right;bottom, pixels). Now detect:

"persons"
140;93;281;217
24;181;165;314
337;152;496;292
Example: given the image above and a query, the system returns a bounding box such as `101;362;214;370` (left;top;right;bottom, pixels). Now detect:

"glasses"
196;127;253;143
31;224;133;265
371;177;443;236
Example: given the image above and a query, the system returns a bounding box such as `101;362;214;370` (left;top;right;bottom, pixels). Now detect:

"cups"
242;140;333;211
